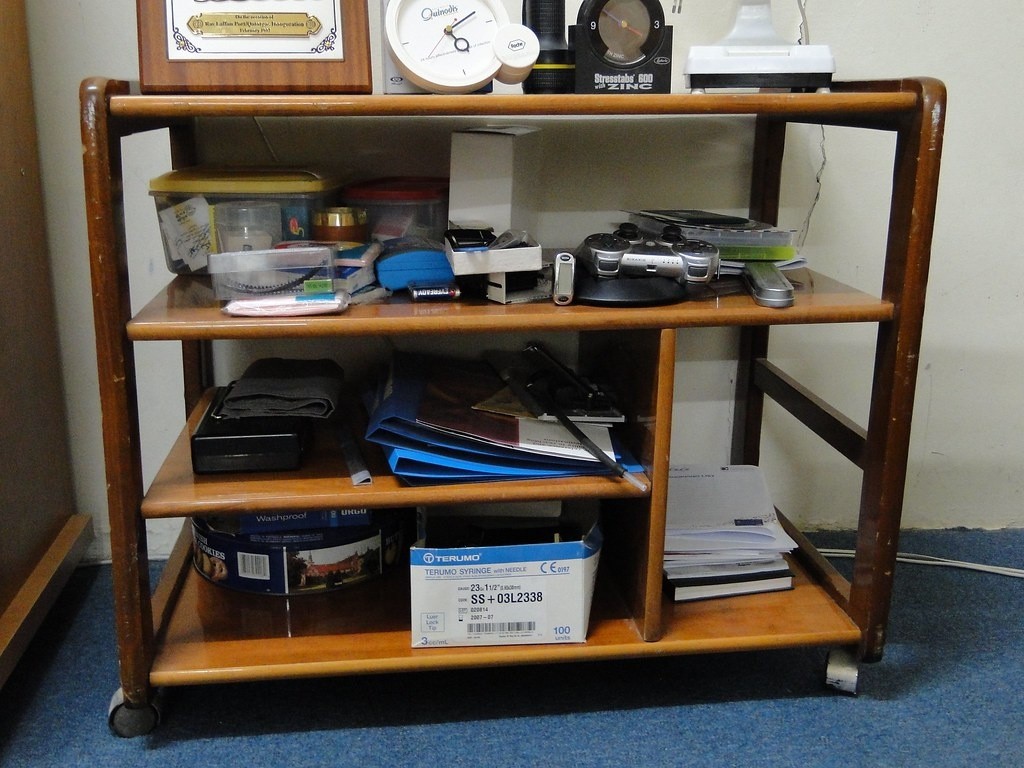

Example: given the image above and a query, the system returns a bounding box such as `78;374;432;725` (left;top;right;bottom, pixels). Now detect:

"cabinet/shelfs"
78;74;947;736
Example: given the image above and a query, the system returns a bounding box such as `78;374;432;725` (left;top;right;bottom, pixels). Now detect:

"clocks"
385;0;541;95
568;0;673;93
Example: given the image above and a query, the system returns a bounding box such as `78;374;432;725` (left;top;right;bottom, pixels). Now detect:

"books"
480;350;625;422
662;465;800;601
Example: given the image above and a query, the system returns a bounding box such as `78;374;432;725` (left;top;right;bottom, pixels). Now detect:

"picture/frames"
136;0;372;97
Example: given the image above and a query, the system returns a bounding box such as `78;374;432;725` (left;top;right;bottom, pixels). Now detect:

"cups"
310;206;369;242
213;201;281;253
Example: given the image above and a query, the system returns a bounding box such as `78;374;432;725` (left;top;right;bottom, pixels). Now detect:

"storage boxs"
408;500;605;648
206;245;338;300
374;237;453;289
148;168;340;278
191;385;314;470
191;508;403;594
446;124;544;241
445;233;542;276
347;181;448;250
